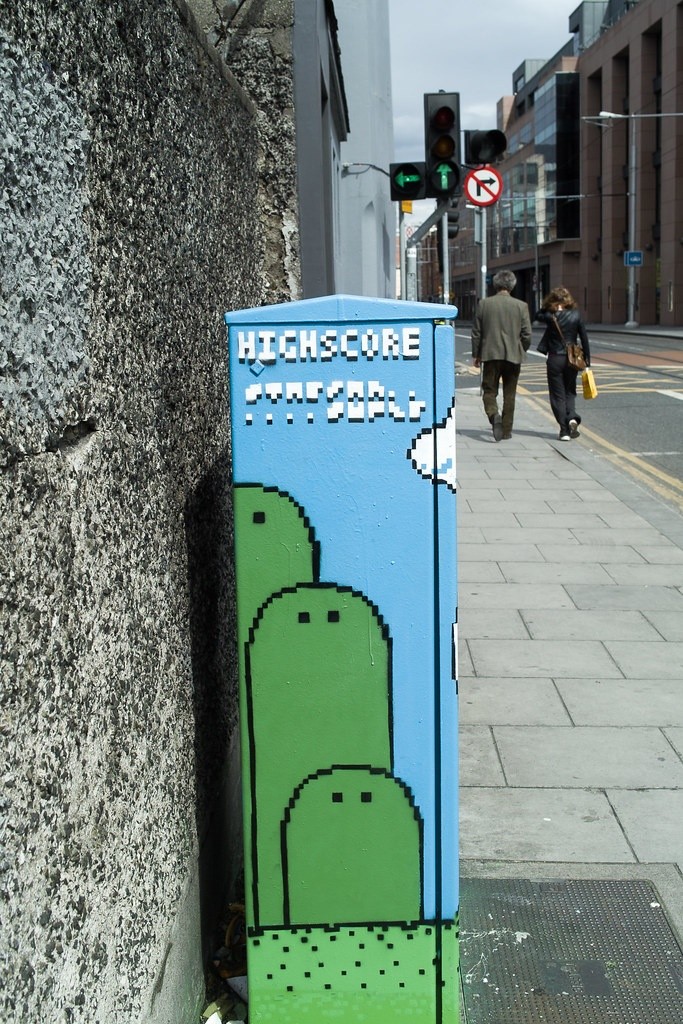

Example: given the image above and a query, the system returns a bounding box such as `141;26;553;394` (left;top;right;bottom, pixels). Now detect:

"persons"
535;287;590;441
471;270;532;440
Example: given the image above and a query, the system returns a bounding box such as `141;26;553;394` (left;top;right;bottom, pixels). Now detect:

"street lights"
600;112;683;119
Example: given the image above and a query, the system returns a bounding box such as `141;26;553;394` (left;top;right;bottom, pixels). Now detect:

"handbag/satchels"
581;367;598;400
568;344;586;371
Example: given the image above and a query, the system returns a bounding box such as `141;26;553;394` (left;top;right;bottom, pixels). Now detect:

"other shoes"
569;419;580;438
502;434;512;439
491;414;503;441
561;435;571;440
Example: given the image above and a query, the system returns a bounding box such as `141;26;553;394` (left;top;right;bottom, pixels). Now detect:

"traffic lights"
446;199;460;239
465;129;507;164
389;162;425;202
424;92;462;198
